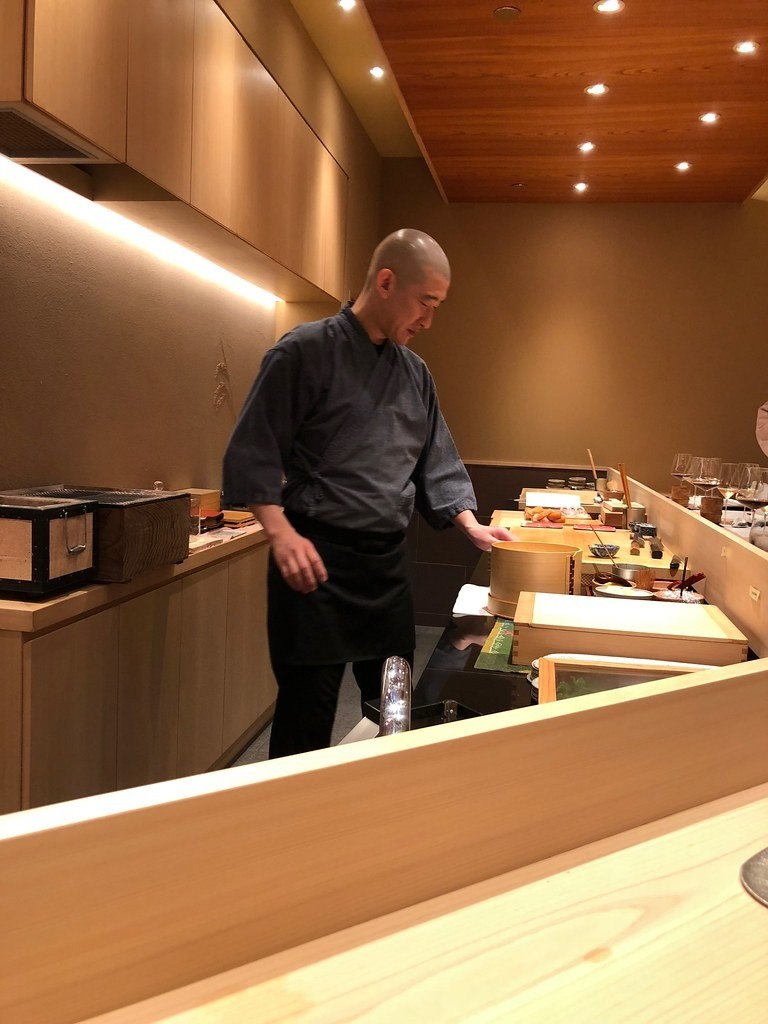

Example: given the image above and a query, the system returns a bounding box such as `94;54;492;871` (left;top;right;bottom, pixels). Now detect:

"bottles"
531;676;539;705
530;658;539;680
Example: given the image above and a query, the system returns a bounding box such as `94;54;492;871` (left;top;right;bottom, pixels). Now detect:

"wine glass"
717;463;739;526
733;467;768;528
761;471;768;527
738;463;759;511
693;458;721;496
686;457;704;510
671;453;693;486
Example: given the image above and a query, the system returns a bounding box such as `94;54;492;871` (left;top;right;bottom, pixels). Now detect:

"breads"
524;506;565;523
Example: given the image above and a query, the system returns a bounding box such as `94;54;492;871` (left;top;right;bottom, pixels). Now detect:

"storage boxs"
17;487;193;585
0;495;100;604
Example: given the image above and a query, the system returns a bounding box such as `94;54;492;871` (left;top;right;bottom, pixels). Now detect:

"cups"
701;497;723;523
627;507;647;530
672;484;691;508
629;521;656;537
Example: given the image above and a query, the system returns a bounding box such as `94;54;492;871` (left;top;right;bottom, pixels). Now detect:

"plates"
654;590;704;604
592;579;654;600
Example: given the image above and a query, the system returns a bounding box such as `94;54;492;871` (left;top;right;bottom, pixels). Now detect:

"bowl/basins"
612;563;650;581
588;544;620;559
604;489;624;501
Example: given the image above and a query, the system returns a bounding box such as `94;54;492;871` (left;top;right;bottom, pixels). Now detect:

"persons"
225;229;520;759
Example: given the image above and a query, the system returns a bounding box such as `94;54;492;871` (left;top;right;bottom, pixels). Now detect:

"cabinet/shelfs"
0;0;374;305
0;541;278;816
